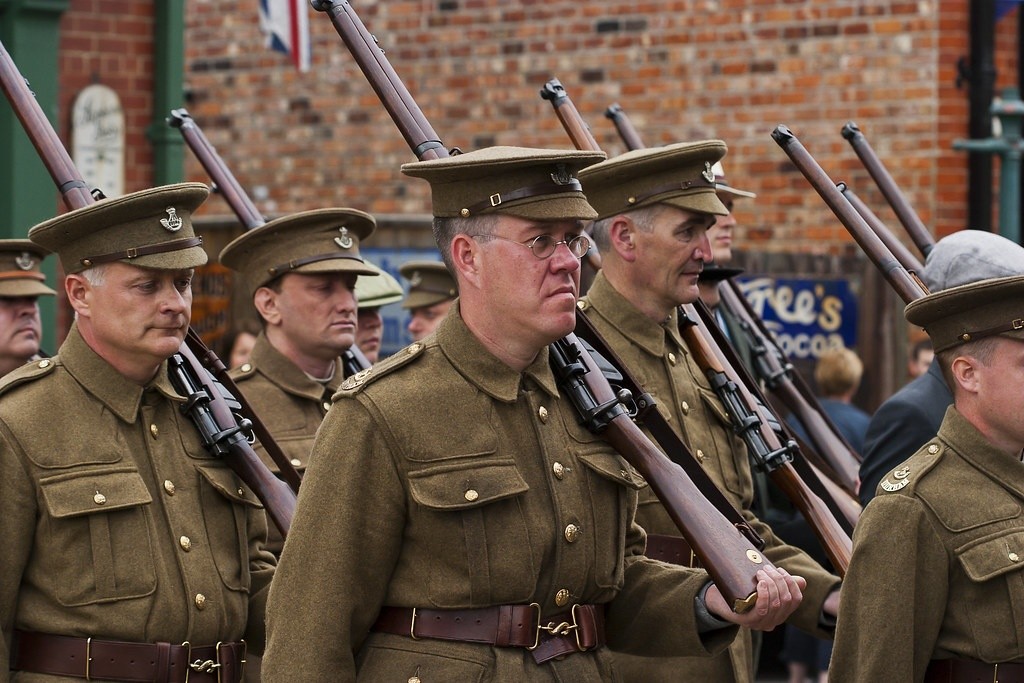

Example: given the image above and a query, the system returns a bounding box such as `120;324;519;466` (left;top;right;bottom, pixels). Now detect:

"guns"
161;103;375;381
539;77;856;577
308;0;781;613
767;119;926;308
603;100;869;501
837;121;937;256
840;181;927;282
0;44;305;542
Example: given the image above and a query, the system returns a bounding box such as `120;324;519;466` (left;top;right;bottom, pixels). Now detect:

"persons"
906;324;934;377
221;208;376;683
859;230;1024;517
697;259;746;320
0;181;277;683
697;161;861;682
0;238;58;379
826;274;1024;683
574;139;843;683
354;259;404;365
261;147;806;683
786;348;871;683
399;262;460;343
219;326;257;371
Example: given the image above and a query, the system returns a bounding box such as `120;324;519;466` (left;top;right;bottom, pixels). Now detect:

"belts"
5;631;248;683
925;660;1024;683
378;603;608;661
644;534;707;569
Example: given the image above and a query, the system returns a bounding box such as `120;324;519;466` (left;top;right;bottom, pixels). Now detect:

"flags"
257;0;311;74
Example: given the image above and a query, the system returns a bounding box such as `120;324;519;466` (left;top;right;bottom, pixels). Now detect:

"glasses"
471;233;592;259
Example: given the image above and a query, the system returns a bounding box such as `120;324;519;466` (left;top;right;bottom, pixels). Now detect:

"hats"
31;180;210;268
218;207;380;291
707;158;755;198
397;261;457;307
399;143;608;217
925;230;1024;291
0;239;57;296
904;274;1024;352
578;139;729;221
353;258;405;306
